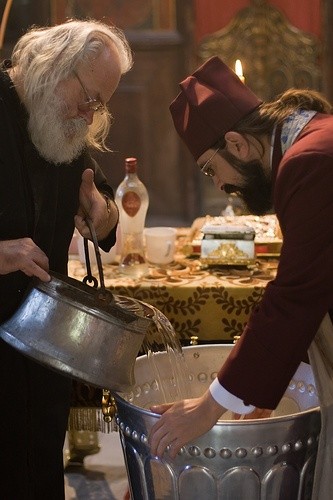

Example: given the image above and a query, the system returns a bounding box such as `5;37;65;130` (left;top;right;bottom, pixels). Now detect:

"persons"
147;55;333;463
0;16;134;500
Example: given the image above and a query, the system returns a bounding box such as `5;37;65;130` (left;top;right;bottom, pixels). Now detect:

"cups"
145;227;175;264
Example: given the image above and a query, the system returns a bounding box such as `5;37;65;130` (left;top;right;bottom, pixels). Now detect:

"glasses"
195;143;225;179
76;69;101;114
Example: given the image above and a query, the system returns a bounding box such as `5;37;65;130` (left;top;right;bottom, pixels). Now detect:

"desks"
68;225;284;341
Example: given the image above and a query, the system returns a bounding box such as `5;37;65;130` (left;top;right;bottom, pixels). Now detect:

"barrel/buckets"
0;214;153;390
114;344;323;500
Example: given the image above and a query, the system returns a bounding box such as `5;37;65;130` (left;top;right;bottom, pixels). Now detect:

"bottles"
233;336;240;344
189;336;199;346
113;158;149;274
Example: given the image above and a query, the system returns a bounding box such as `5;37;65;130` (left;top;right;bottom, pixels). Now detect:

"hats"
167;55;264;162
